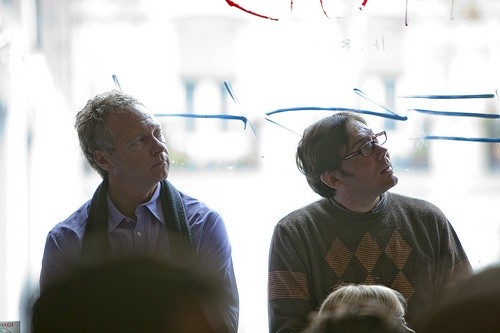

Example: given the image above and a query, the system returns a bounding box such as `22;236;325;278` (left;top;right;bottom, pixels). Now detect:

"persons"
267;112;474;333
39;89;239;333
318;284;417;333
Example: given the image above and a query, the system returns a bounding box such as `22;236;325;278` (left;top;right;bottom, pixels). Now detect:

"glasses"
339;131;387;163
